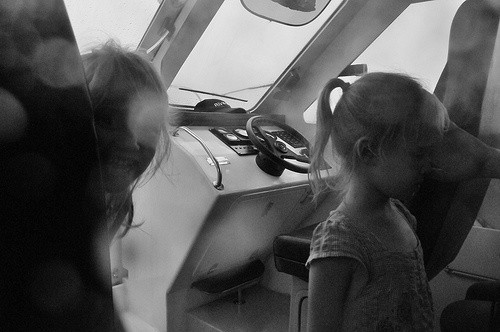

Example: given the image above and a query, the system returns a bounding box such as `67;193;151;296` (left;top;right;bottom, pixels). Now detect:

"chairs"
0;0;173;332
273;0;499;282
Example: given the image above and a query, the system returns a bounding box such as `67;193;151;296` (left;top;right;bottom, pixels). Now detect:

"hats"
194;99;246;113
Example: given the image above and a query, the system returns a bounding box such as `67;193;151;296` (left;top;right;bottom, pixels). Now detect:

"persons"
80;37;172;248
305;71;451;332
